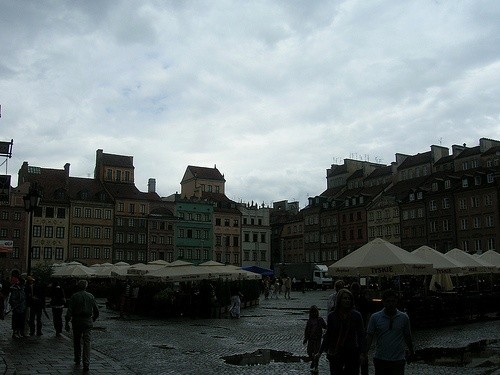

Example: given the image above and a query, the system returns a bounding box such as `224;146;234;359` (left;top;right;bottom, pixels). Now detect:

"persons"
328;275;443;329
328;289;366;375
0;275;66;339
64;280;100;371
303;305;327;373
301;274;305;293
366;289;415;375
228;291;241;319
314;331;336;375
258;271;297;299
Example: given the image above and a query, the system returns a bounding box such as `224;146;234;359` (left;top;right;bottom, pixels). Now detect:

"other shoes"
83;365;89;371
29;333;34;335
12;334;20;339
74;363;80;366
16;333;23;337
37;332;43;335
25;334;30;336
310;358;316;369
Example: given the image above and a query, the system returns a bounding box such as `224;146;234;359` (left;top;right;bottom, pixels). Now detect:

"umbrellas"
51;259;262;281
477;250;500;273
328;237;433;275
411;245;472;276
240;265;274;276
429;274;454;291
443;248;497;272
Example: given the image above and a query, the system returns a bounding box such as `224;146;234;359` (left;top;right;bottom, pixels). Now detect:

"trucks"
278;263;333;290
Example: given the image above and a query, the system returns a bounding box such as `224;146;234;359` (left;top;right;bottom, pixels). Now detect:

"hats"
27;276;35;282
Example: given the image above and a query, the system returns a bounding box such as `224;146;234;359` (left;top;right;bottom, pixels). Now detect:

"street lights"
23;190;42;288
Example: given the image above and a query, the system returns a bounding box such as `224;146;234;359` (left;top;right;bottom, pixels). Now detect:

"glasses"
389;319;394;330
